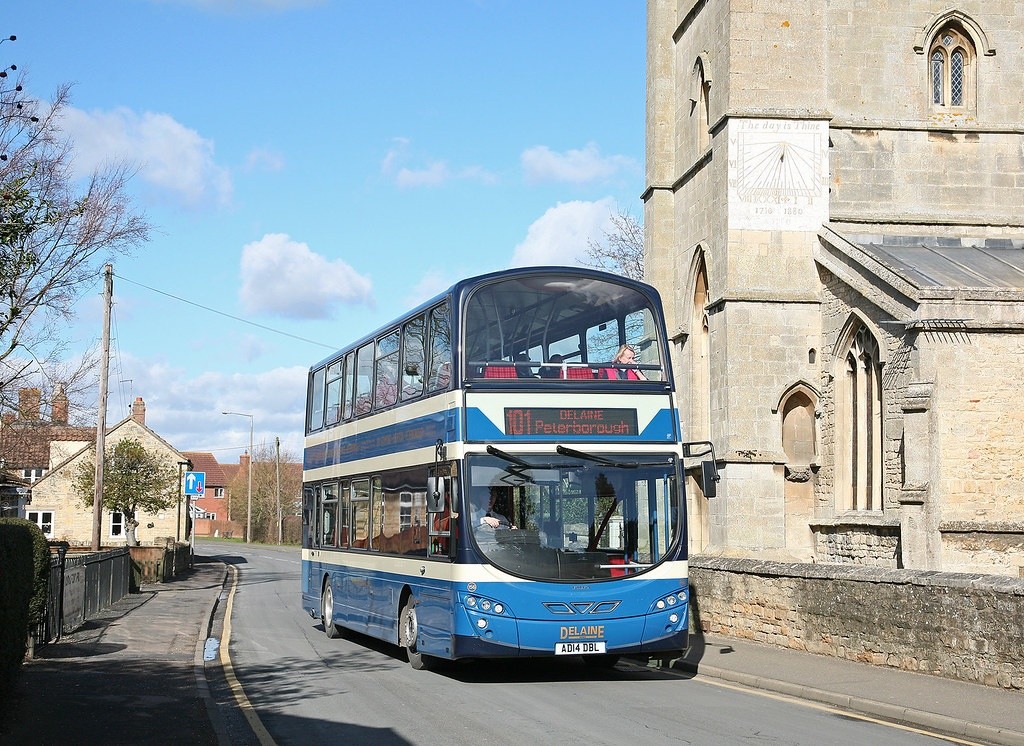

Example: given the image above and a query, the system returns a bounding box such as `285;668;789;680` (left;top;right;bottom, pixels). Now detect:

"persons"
600;344;649;380
538;354;564;379
513;354;535;378
470;486;519;532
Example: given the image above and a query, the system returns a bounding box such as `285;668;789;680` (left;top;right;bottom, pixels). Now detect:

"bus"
301;267;719;671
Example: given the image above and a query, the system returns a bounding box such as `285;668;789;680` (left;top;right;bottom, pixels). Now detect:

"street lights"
220;411;254;543
